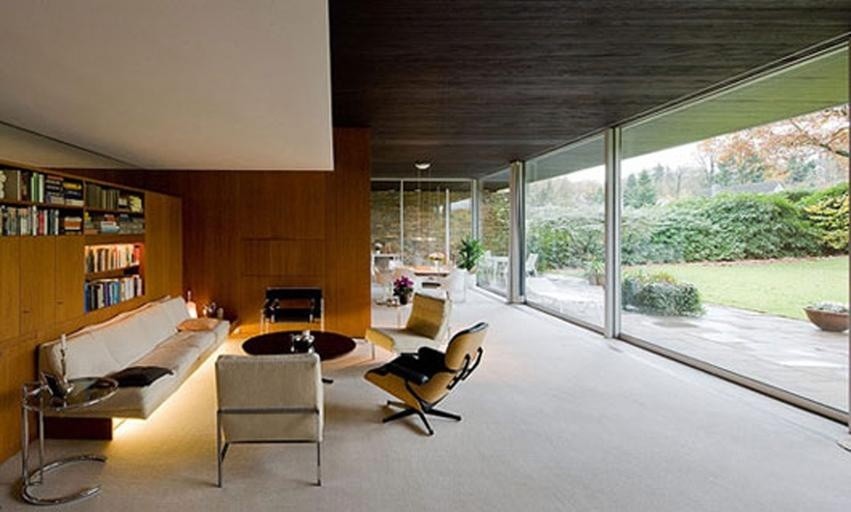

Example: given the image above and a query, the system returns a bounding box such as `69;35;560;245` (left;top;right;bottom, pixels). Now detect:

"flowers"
428;251;446;261
393;276;415;296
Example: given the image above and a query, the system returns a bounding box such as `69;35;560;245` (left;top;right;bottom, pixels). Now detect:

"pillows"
112;365;174;388
176;319;221;331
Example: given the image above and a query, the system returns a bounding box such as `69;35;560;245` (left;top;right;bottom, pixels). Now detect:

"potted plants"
803;300;851;334
457;235;489;287
585;259;606;286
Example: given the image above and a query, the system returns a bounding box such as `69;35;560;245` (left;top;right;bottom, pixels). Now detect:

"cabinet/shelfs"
0;237;85;343
0;158;147;237
84;242;146;312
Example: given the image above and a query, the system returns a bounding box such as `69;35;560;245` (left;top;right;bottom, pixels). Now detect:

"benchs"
37;293;231;443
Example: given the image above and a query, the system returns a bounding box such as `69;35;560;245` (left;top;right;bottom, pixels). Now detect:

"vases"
434;260;441;271
399;294;409;304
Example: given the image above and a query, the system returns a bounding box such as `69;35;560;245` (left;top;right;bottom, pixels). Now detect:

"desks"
373;294;411;328
371;252;395;275
20;376;120;506
399;264;450;290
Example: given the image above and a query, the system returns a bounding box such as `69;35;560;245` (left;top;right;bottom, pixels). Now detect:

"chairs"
213;352;324;489
490;253;541;286
258;285;327;335
365;291;454;362
365;321;490;436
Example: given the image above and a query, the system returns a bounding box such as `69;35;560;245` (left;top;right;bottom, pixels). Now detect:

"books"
85;240;145;310
0;170;146;237
